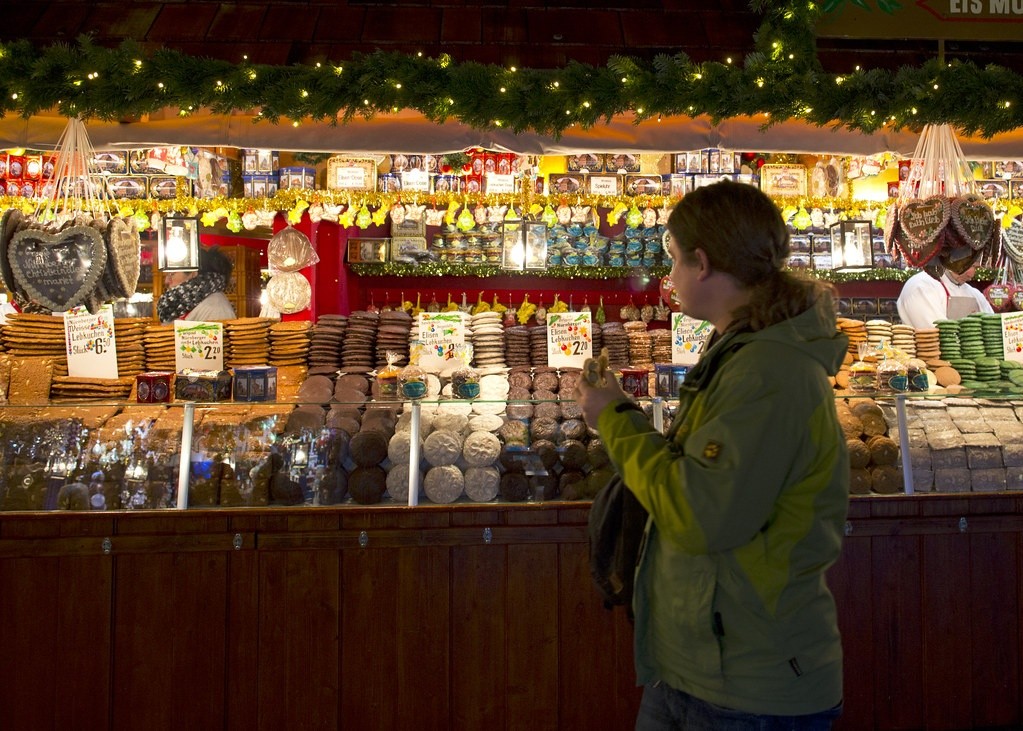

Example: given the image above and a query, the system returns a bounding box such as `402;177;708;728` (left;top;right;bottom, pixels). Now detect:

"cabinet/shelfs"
0;196;1023;299
106;239;165;321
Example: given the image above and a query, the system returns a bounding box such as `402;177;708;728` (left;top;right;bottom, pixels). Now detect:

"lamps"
159;177;200;274
827;157;874;273
500;167;549;271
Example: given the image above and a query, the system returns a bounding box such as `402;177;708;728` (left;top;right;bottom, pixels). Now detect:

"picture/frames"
761;164;807;196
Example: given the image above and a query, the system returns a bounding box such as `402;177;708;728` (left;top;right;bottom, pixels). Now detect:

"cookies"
831;397;1023;493
0;312;671;512
826;311;1023;400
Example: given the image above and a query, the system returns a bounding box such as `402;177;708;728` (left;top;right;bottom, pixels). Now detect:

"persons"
896;252;995;331
156;243;238;321
569;177;850;731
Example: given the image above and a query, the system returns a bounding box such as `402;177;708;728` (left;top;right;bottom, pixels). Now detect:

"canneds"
432;215;503;266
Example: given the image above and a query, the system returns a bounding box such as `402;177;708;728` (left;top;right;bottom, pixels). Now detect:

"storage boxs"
349;206;429;264
376;152;760;195
655;362;694;397
619;366;651;397
233;364;279;402
0;148;316;199
887;159;1023;199
785;221;900;269
207;242;261;321
176;368;233;403
136;371;174;404
